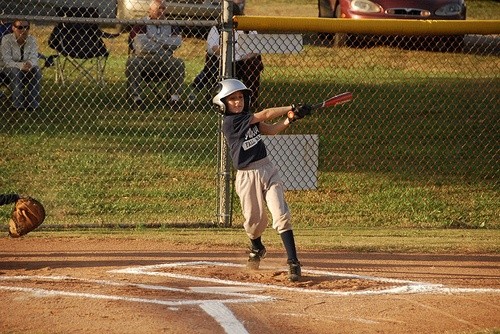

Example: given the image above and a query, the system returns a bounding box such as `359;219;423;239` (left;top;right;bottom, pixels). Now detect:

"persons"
0;193;28;206
1;19;39;111
212;79;312;283
205;2;261;98
125;0;186;105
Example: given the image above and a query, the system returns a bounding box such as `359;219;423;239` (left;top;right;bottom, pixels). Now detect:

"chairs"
0;22;58;97
128;23;173;106
51;6;109;85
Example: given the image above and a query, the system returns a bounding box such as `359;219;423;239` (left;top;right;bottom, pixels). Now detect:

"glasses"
13;23;30;31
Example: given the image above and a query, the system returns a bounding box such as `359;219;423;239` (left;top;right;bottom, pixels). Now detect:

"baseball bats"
287;91;354;119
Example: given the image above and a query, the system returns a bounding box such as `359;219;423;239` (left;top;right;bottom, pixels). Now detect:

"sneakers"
247;245;266;269
287;259;302;281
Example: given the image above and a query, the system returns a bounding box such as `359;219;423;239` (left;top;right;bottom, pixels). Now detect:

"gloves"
287;104;311;124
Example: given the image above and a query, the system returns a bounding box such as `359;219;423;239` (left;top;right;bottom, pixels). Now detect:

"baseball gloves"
7;197;46;237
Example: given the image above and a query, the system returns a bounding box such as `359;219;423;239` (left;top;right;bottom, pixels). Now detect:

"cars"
327;0;467;52
116;0;246;32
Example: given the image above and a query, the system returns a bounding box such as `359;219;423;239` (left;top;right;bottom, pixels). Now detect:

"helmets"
213;79;254;115
